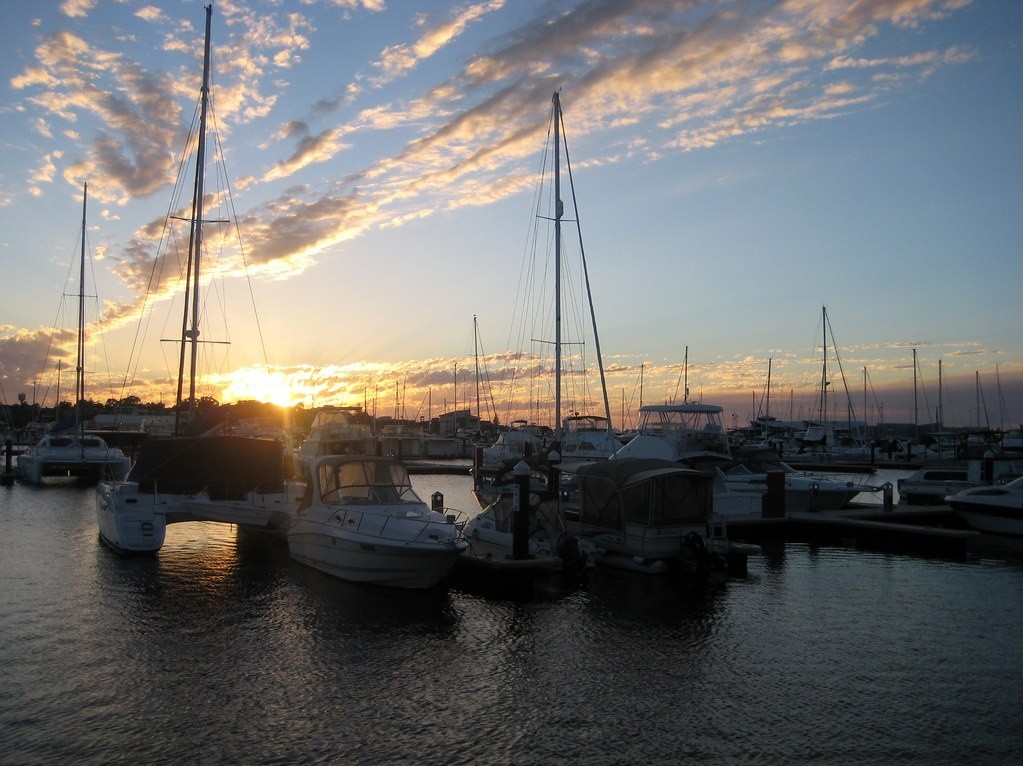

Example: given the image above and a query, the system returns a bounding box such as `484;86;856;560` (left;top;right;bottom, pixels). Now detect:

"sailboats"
18;3;1023;589
